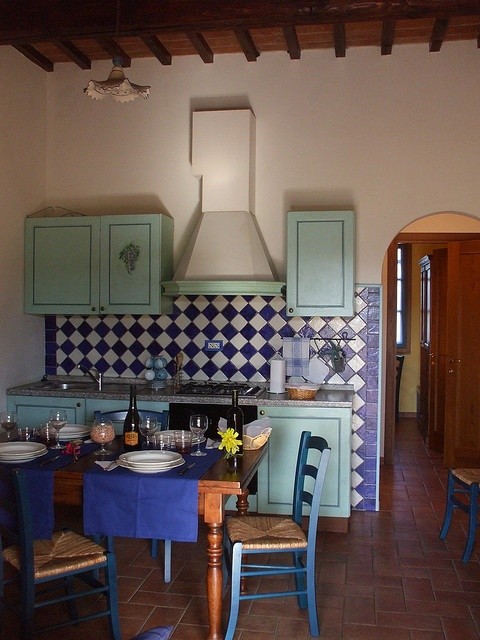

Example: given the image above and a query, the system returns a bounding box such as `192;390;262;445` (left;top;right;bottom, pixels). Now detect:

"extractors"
160;211;287;297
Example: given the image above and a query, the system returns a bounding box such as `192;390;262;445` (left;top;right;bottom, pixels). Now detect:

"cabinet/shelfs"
5;395;170;436
285;210;357;317
23;214;174;315
418;248;446;453
258;403;351;518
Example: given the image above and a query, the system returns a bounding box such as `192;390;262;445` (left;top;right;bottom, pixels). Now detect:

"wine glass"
48;408;68;450
138;415;158;450
38;423;56;444
190;415;208;457
89;419;116;456
0;411;18;442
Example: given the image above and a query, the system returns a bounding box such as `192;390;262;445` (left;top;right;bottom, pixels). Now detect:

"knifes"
39;453;63;467
178;462;196;475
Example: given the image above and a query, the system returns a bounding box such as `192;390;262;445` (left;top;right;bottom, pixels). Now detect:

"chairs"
93;408;168;558
0;464;124;640
440;467;479;565
395;353;405;429
222;431;331;640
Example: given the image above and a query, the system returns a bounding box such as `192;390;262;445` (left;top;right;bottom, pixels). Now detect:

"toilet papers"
270;358;286;393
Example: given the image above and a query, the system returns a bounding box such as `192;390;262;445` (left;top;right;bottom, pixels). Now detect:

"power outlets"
205;340;223;351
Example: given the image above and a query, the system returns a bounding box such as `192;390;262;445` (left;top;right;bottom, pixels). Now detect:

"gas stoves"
175;381;266;398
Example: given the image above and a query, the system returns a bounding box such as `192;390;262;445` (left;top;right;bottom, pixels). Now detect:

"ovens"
169;403;258;494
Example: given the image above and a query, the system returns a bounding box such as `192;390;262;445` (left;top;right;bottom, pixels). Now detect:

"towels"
282;336;311;379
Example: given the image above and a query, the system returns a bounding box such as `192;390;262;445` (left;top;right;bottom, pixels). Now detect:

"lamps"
81;53;153;103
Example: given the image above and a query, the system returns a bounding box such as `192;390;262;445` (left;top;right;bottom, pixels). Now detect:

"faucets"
78;364;102;390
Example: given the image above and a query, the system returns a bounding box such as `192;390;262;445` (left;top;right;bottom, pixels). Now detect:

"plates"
148;430;207;447
0;449;49;464
0;441;47;455
119;449;183;467
50;423;91;442
116;458;186;473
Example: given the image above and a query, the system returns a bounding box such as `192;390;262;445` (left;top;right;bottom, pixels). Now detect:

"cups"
157;369;168;380
144;368;155;380
154;434;172;451
153;357;163;369
17;425;31;441
159;357;168;367
145;358;154;369
173;430;192;455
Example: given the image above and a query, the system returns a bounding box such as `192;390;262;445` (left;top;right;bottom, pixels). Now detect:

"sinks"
29;380;144;396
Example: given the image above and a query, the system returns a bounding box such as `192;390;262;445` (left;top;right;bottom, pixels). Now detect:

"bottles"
226;390;244;455
122;384;142;452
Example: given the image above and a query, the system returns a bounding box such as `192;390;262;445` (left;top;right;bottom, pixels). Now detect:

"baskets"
286;388;317;399
243;428;272;451
